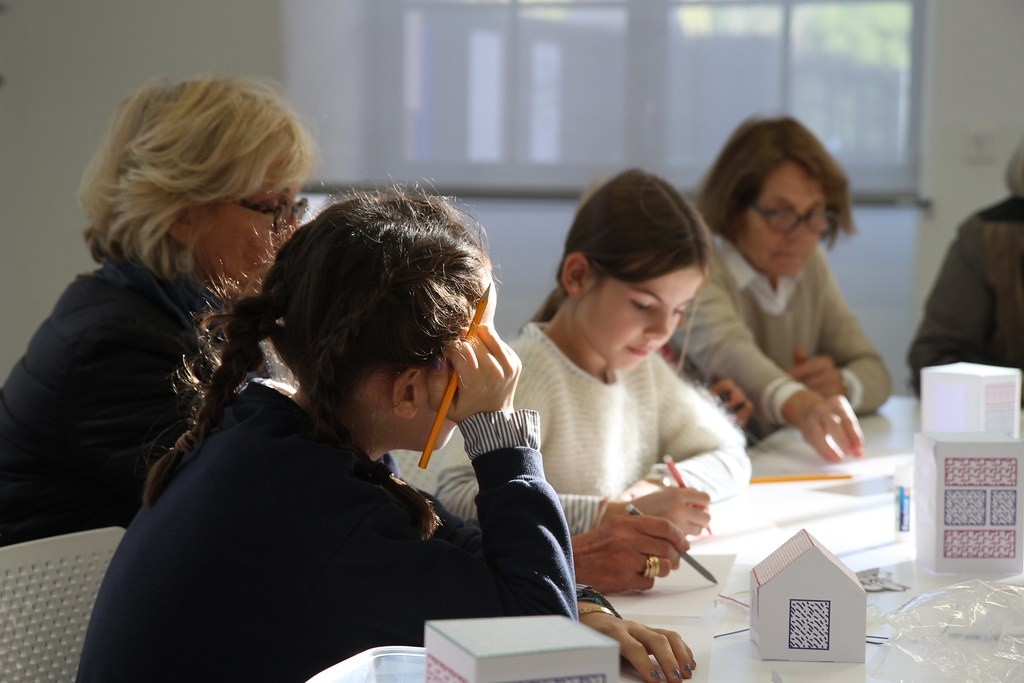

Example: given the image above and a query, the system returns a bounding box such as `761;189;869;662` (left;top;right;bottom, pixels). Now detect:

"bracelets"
578;606;615;617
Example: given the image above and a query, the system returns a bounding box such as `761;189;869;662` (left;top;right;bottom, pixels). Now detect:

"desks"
602;394;1023;683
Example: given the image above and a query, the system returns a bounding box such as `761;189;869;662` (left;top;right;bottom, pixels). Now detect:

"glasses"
751;197;836;235
233;199;308;234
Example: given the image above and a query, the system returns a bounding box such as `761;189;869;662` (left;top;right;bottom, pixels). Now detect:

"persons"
71;188;697;683
904;150;1024;407
431;168;752;537
0;74;690;596
660;114;893;462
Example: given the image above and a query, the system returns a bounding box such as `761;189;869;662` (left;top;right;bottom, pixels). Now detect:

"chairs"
0;527;127;683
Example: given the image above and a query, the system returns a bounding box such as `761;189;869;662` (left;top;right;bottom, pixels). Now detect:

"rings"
644;555;660;577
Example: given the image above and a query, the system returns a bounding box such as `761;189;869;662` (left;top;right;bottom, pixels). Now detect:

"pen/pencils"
752;475;852;484
662;454;713;534
626;505;719;584
418;281;493;470
